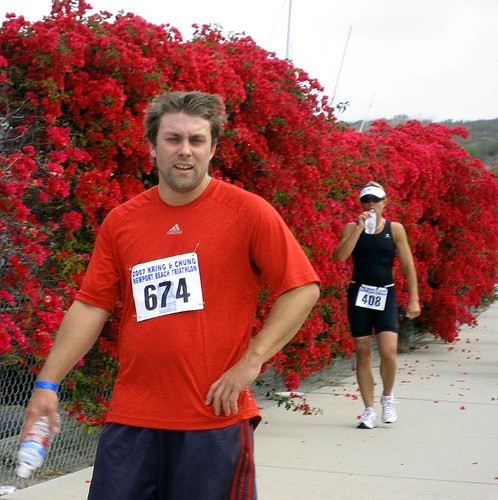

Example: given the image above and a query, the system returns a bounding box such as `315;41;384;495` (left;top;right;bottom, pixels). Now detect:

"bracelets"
34;381;59;393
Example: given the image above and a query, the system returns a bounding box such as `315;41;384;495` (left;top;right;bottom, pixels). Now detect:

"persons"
335;181;420;429
22;91;321;500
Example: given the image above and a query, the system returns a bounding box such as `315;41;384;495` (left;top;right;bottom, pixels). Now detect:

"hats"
359;188;386;199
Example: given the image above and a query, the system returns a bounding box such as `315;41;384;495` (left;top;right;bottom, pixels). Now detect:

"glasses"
360;195;384;204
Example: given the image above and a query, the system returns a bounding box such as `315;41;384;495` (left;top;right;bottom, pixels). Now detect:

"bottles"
17;412;61;478
364;208;376;234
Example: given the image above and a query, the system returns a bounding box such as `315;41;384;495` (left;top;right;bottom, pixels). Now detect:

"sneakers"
380;394;398;423
356;407;378;429
